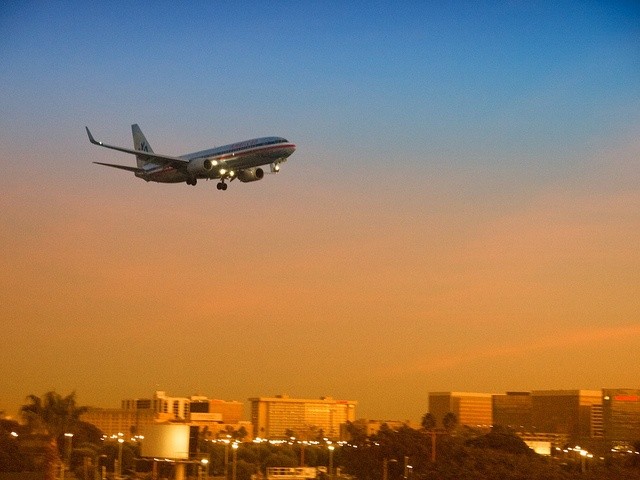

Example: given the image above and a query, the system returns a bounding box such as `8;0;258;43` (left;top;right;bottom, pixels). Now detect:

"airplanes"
84;122;298;192
